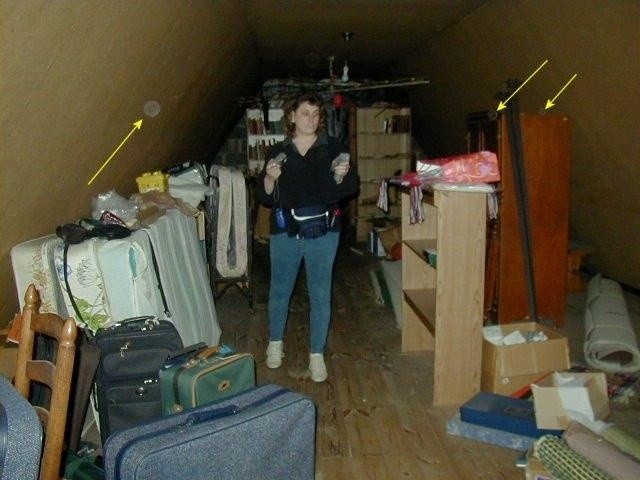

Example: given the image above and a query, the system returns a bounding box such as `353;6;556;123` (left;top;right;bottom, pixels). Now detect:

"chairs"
0;280;78;480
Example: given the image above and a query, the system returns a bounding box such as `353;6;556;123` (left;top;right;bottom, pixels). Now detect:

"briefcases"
102;385;315;479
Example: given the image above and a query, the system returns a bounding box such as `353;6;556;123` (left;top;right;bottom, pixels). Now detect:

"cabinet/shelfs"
467;105;571;331
398;186;489;411
346;105;415;243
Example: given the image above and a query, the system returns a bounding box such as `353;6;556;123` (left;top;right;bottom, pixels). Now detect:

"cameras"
272;152;288;166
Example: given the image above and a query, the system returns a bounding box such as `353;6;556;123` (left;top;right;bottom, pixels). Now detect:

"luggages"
94;316;184;446
159;345;255;416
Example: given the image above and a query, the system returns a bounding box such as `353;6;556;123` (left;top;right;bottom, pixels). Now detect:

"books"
79;217;101;232
246;115;286;190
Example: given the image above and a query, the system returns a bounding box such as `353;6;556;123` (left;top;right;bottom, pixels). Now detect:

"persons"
256;88;361;383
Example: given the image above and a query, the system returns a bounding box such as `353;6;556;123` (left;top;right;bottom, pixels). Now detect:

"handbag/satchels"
287;204;328;240
415;151;501;184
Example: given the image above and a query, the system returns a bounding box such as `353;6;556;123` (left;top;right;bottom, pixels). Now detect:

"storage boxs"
478;320;611;433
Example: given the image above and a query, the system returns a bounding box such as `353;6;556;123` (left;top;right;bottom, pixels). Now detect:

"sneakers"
309;352;328;383
267;341;283;369
